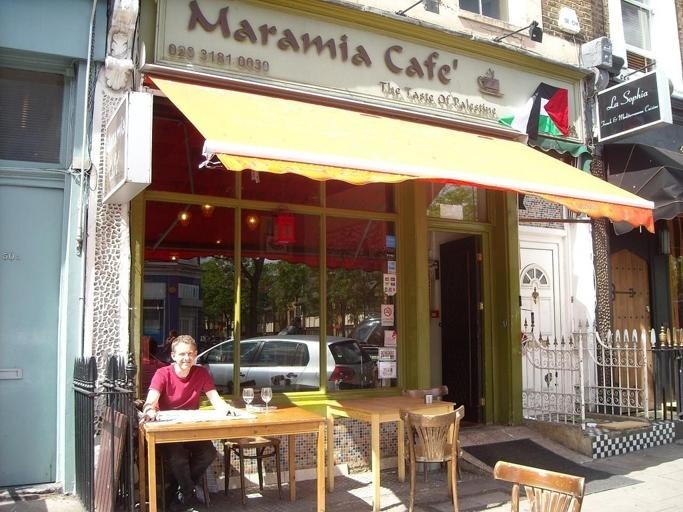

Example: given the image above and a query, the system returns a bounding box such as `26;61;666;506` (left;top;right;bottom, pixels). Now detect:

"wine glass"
261;387;272;414
242;387;254;414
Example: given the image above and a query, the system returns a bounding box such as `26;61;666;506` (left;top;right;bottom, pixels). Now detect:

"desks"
138;404;325;511
327;396;456;511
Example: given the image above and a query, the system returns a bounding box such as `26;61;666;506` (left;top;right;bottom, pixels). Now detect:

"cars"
348;319;397;386
194;335;376;395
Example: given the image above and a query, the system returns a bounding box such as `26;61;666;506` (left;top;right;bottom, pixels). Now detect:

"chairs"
132;450;210;510
492;459;586;510
399;404;467;512
401;386;463;482
221;401;283;503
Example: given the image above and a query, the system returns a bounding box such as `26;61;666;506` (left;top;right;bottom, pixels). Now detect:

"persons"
280;317;304;335
142;334;242;512
165;330;179;359
